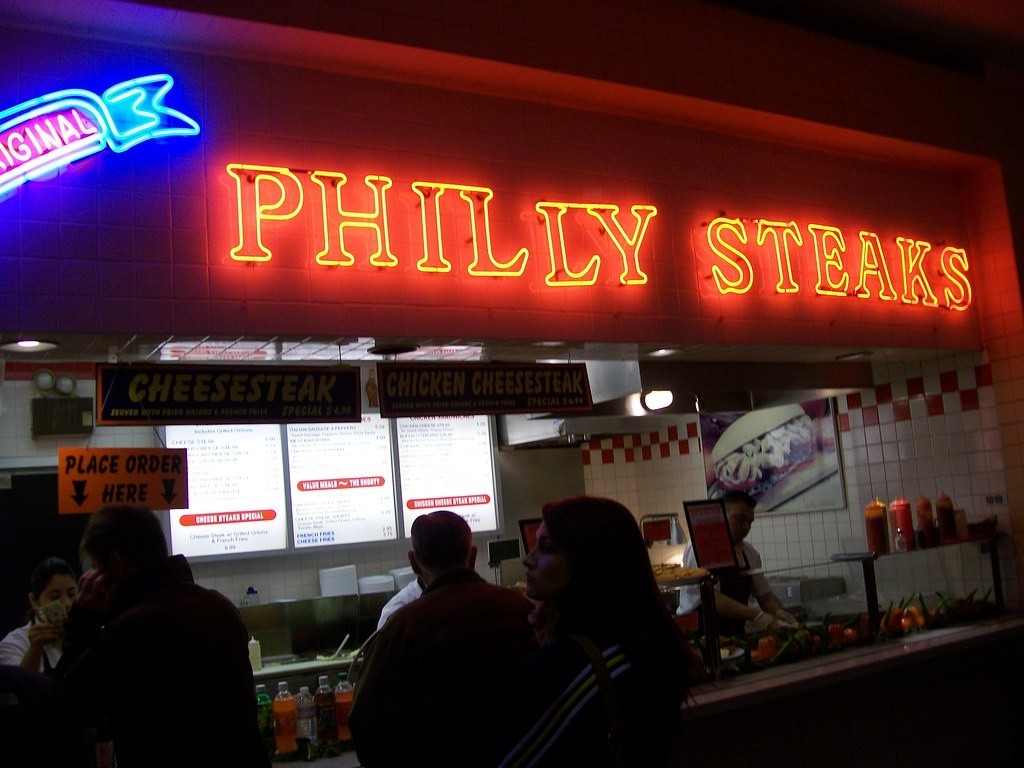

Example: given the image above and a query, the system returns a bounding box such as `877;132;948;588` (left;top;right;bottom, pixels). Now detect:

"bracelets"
753;611;764;624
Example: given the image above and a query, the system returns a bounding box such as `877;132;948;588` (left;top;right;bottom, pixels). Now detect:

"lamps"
0;333;58;354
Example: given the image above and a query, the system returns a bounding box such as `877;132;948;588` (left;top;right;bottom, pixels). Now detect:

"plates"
653;567;709;588
721;647;744;661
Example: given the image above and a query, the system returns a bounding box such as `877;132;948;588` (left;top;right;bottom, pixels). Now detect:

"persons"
60;505;271;768
377;574;424;629
347;510;536;768
675;490;798;639
0;556;97;768
492;496;696;768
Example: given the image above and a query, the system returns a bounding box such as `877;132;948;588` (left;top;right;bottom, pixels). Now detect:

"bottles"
896;529;906;552
296;687;315;744
314;675;338;745
937;489;960;544
916;493;938;549
333;672;354;741
248;635;261;671
274;681;297;754
890;495;915;550
865;497;889;554
256;684;274;756
